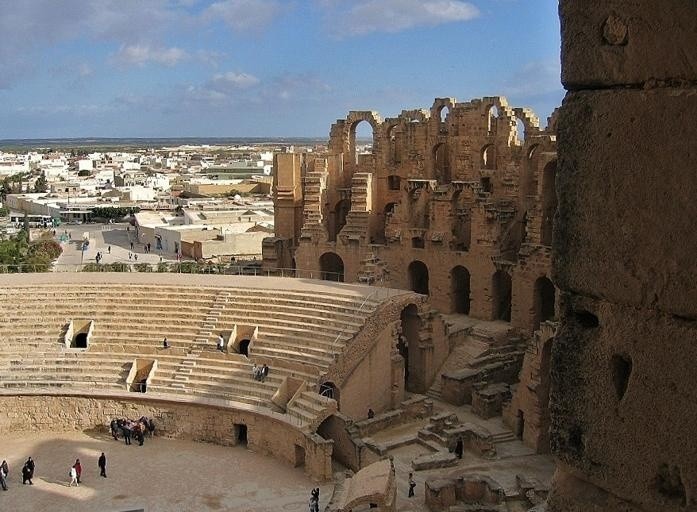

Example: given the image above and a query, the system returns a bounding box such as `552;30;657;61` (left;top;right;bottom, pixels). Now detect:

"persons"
74;460;82;483
455;436;463;459
259;364;269;383
27;458;35;478
0;466;9;491
1;460;8;479
99;452;107;479
22;463;34;485
311;488;320;512
163;340;170;349
367;409;374;419
68;465;79;487
252;364;260;381
52;228;163;264
309;497;316;512
110;417;156;447
218;335;224;353
408;473;416;498
